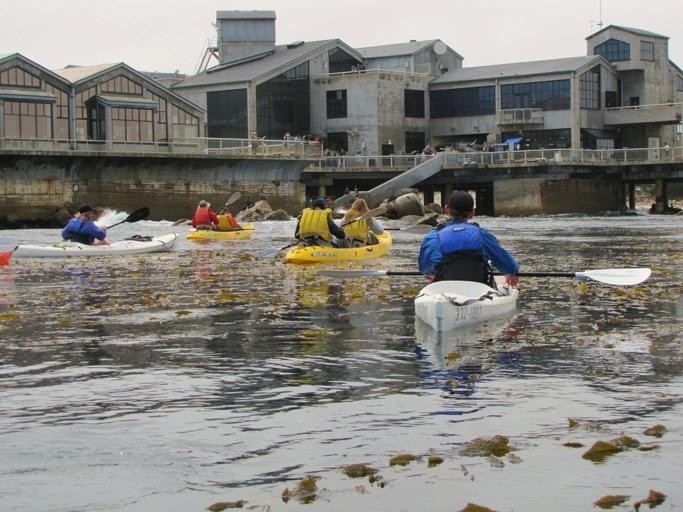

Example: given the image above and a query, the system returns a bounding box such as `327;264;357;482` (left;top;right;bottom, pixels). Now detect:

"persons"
664;142;669;155
418;191;519;291
217;207;242;231
339;199;384;247
350;63;361;74
251;131;494;168
62;205;110;245
295;198;350;247
192;200;220;231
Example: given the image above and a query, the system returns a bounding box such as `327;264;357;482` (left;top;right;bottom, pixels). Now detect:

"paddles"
318;267;652;286
105;207;149;230
366;223;434;235
216;191;241;215
277;207;387;252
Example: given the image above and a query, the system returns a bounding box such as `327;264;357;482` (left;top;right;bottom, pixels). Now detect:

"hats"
311;199;324;209
198;200;211;208
446;192;474;211
79;204;96;213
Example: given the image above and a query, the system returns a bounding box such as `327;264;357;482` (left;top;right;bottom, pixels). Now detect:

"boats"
285;230;392;263
648;207;683;215
13;221;254;258
414;276;519;332
